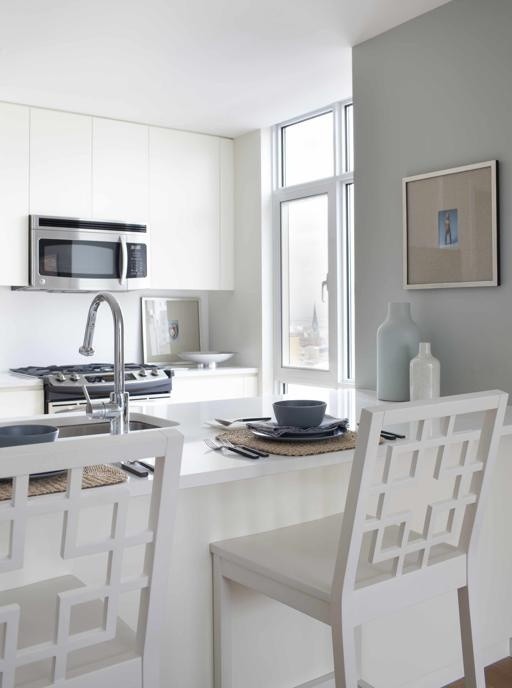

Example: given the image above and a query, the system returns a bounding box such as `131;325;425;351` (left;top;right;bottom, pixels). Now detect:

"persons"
444;214;452;245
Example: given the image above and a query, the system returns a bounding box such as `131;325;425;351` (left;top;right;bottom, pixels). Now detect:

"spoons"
215;416;271;427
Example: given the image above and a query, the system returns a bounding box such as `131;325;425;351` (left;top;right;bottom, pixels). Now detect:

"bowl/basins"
0;425;59;447
272;400;326;424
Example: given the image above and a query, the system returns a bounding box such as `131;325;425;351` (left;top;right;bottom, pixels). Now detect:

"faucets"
75;293;131;435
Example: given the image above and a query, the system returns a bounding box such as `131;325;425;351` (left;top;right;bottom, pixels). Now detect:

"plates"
246;421;344;442
28;470;66;479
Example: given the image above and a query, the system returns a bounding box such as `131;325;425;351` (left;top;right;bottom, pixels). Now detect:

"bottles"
377;301;421;403
409;342;441;403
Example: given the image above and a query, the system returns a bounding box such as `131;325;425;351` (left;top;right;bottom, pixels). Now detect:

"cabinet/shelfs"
0;101;235;290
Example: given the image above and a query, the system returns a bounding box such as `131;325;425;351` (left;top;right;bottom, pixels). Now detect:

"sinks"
57;412;178;436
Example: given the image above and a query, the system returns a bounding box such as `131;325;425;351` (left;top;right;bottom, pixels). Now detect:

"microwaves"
28;216;149;288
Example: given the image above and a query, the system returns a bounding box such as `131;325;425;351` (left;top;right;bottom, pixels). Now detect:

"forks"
206;439;258;460
219;437;268;457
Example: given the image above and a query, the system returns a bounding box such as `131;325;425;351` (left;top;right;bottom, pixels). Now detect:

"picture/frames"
141;296;202;367
402;160;500;289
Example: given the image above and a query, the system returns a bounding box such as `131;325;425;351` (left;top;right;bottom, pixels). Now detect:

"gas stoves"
12;362;157;374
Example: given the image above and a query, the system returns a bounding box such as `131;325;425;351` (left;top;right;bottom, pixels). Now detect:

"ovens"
45;387;170;413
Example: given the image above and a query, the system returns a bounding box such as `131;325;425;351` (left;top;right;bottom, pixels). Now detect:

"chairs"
0;427;185;686
208;389;509;686
